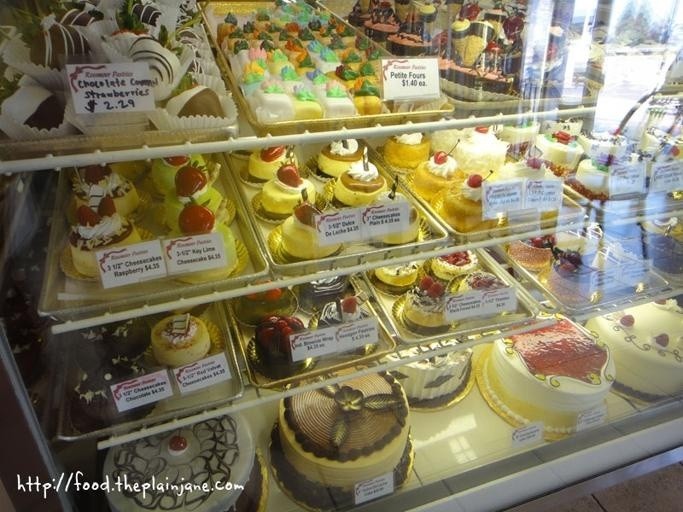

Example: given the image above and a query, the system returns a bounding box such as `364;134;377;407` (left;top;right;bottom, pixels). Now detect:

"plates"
57;78;683;434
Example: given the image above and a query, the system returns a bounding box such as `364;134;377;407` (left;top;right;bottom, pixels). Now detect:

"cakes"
485;313;617;434
640;215;683;243
247;138;419;262
268;358;413;510
375;336;473;407
67;154;238;282
509;232;560;269
374;250;509;336
644;231;683;272
67;314;211;434
346;0;567;94
495;120;683;202
586;300;683;402
101;402;262;512
0;1;224;129
384;126;561;232
545;251;603;302
215;2;389;125
237;271;365;374
3;219;51;382
